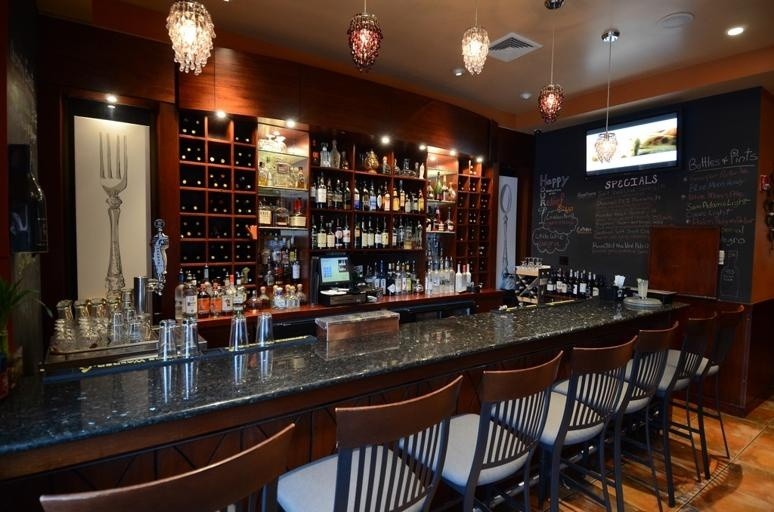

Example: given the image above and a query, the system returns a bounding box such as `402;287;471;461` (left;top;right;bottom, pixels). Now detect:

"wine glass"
520;257;543;272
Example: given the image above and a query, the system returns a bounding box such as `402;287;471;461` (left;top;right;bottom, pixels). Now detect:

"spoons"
498;184;513;278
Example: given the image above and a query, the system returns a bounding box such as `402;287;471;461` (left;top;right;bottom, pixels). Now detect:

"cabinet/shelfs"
157;43;503;326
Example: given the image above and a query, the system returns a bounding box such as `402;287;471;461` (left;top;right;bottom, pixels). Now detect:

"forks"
98;133;129;292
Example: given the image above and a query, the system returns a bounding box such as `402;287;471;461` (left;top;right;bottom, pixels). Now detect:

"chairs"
396;349;565;511
277;373;462;511
41;426;299;512
618;310;717;482
547;319;681;510
647;304;745;479
488;334;639;512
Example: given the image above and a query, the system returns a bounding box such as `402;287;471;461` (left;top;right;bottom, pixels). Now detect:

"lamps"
461;1;489;78
538;0;567;125
164;0;216;78
346;0;384;77
595;31;620;163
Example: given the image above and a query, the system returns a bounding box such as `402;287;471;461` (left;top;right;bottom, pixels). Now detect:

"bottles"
176;131;487;320
515;276;537;298
541;267;600;299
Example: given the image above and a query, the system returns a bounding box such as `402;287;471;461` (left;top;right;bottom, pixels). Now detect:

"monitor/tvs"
582;109;684;180
315;252;353;295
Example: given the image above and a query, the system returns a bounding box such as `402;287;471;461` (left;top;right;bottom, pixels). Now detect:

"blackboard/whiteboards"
530;87;762;304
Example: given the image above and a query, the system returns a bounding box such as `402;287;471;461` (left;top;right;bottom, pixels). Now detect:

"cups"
229;316;248;351
157;317;199;356
255;314;275;345
51;291;152;350
160;361;201;401
230;354;248;386
257;350;275;382
637;279;648;301
